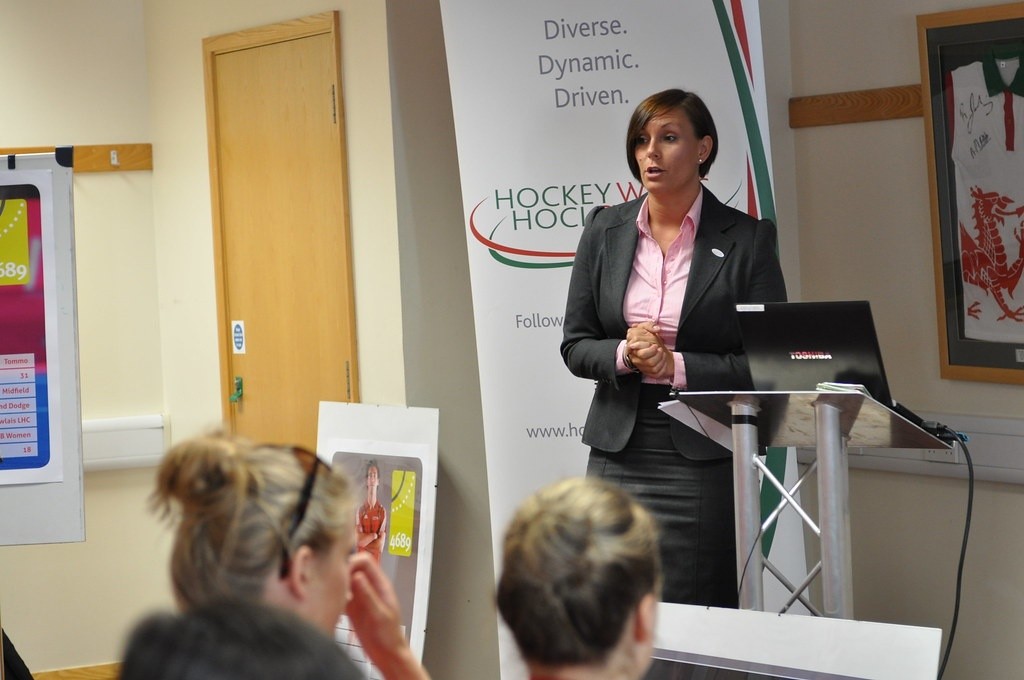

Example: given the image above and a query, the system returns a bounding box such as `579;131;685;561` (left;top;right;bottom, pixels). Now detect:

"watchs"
618;345;637;372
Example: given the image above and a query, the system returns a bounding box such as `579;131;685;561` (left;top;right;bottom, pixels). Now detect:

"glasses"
278;448;332;580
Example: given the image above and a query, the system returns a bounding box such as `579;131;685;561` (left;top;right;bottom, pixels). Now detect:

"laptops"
734;299;954;448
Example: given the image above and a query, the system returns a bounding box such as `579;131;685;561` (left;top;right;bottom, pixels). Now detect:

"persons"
559;87;786;609
113;430;436;680
350;459;389;566
496;478;660;680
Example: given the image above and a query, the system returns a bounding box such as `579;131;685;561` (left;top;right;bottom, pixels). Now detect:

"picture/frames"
915;2;1024;386
